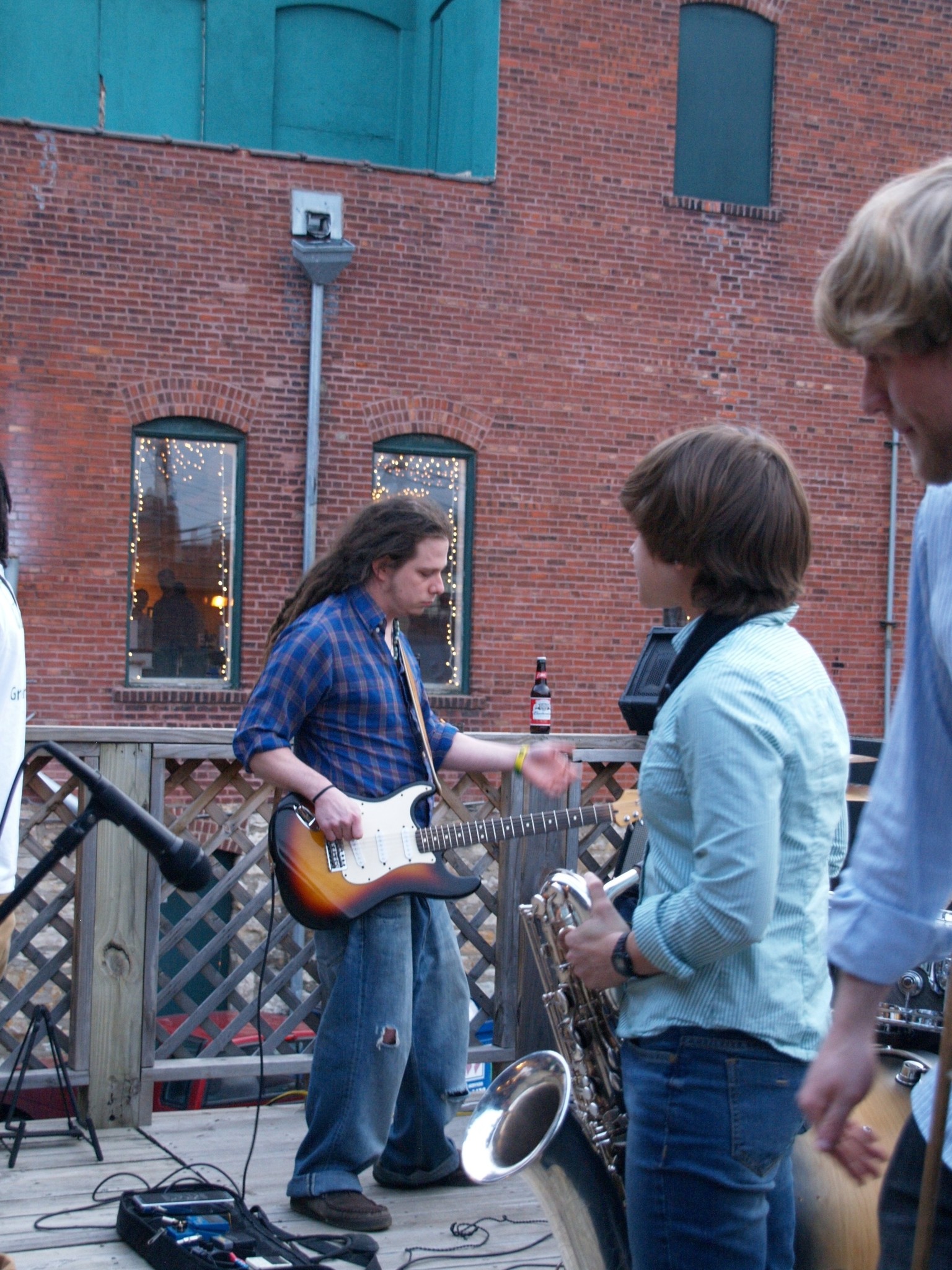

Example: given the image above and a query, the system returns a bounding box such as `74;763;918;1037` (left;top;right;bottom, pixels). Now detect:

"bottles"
529;657;551;734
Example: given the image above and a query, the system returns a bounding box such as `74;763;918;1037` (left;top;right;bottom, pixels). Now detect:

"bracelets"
312;784;338;803
513;743;529;774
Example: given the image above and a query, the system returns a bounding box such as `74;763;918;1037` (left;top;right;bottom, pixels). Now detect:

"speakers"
618;627;687;736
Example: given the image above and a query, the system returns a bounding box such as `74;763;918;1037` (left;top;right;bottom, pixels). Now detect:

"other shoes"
288;1187;393;1232
371;1148;484;1188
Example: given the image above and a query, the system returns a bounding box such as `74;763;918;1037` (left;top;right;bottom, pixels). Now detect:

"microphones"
48;739;212;892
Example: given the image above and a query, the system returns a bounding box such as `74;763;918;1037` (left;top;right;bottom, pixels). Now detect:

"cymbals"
847;753;880;765
845;781;875;803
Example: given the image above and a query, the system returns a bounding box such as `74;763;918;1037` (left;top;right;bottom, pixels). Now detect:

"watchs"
611;930;656;982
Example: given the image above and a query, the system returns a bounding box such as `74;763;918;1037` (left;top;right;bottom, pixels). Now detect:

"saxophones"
460;864;642;1270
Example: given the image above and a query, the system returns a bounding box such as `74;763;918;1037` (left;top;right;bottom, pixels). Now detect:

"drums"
873;906;952;1037
790;1045;945;1270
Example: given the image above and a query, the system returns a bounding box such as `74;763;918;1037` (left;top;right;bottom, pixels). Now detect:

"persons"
564;422;850;1270
134;569;202;677
232;494;582;1233
791;154;952;1270
0;461;26;982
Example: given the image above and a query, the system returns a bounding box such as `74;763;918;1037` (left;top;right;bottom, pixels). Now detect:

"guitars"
268;780;646;933
